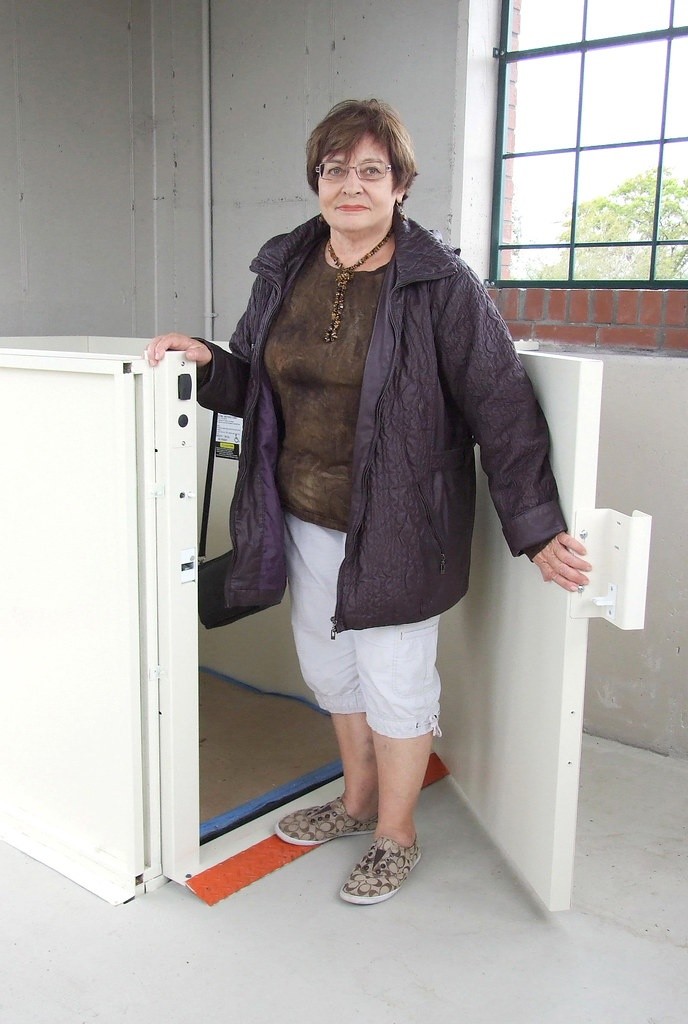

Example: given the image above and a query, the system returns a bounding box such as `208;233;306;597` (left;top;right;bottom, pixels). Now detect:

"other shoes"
276;796;378;846
339;832;422;905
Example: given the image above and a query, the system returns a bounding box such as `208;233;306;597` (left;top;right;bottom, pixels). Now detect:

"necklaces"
323;225;394;342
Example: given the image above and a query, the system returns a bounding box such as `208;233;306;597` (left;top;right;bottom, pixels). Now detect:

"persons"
145;100;591;905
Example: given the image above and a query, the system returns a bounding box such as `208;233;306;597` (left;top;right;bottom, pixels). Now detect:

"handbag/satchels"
198;549;281;629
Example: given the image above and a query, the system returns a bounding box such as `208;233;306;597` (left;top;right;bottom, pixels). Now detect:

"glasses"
315;162;392;180
